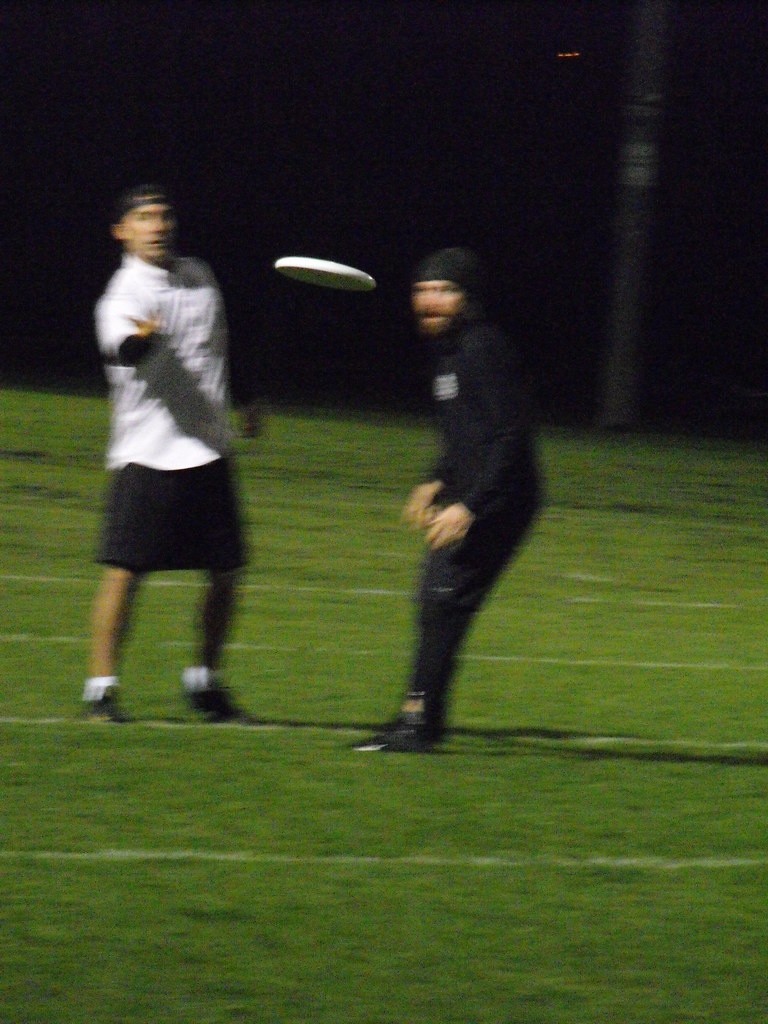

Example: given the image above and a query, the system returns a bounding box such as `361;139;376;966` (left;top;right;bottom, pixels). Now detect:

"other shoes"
81;686;135;722
190;690;239;722
348;713;444;752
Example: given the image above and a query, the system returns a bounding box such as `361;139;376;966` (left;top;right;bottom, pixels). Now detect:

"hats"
117;183;172;219
411;247;481;291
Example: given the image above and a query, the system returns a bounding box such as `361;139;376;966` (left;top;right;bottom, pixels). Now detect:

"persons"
82;186;259;722
358;245;547;750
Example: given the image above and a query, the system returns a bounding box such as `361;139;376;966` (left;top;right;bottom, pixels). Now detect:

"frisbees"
273;256;377;292
